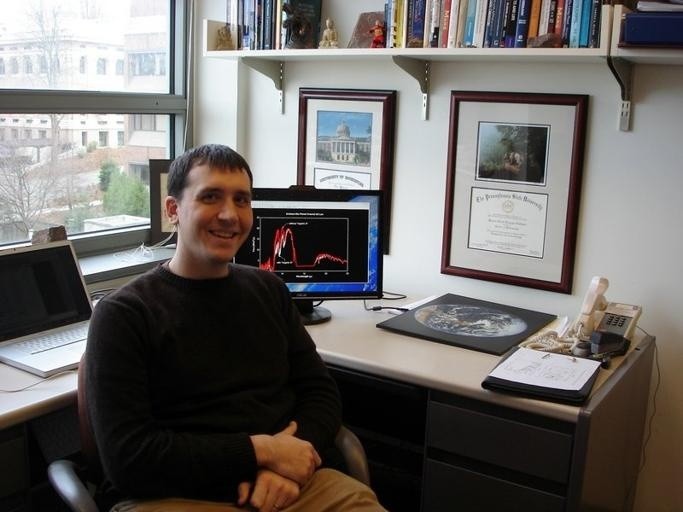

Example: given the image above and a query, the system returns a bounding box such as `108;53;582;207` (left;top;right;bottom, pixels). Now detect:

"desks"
0;296;656;512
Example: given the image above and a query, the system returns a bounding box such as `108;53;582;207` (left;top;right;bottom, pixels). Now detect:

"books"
226;0;683;49
481;345;601;407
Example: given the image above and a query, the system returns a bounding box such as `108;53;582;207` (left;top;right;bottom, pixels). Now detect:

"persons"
85;143;387;512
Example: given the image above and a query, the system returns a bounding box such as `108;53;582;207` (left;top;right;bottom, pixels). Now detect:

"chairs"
47;351;372;512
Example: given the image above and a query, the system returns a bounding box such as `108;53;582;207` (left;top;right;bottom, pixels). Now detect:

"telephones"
573;277;642;357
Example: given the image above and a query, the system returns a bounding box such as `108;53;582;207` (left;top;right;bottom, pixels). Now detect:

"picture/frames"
297;88;396;255
441;90;589;294
150;159;176;245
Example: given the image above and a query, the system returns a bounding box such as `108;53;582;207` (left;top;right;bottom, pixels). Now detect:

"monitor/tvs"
230;188;384;326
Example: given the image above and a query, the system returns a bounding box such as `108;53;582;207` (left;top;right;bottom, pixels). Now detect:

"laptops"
0;240;95;379
